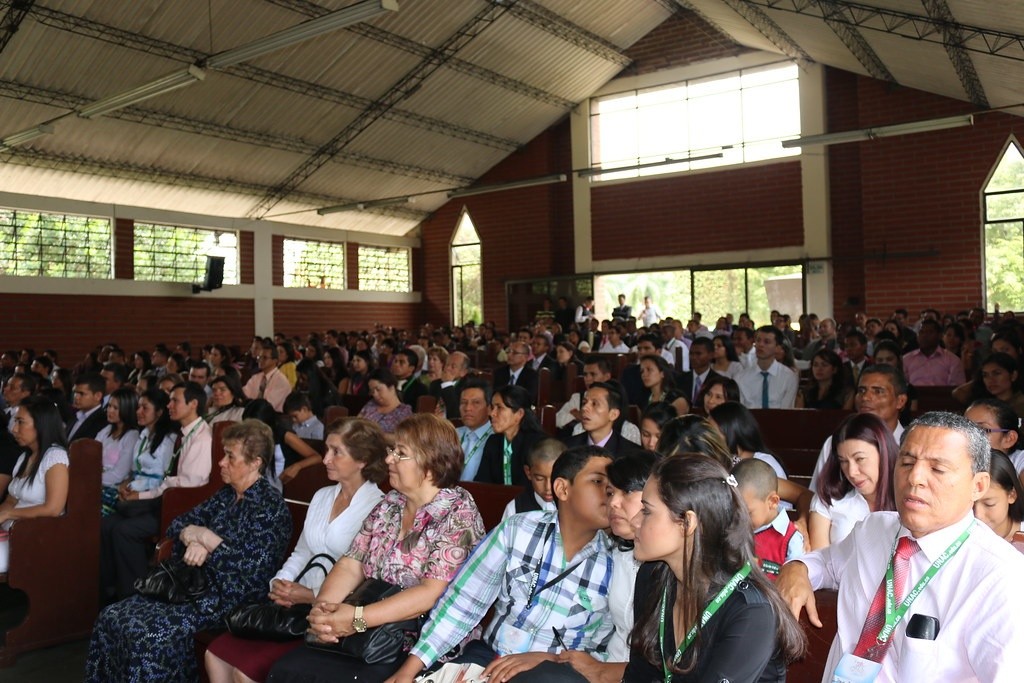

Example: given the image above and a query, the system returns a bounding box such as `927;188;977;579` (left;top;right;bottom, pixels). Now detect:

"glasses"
505;348;527;355
256;355;273;361
385;446;415;462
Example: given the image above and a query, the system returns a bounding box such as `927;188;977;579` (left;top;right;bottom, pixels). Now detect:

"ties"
435;397;445;419
257;375;268;399
694;375;702;396
853;537;922;662
510;374;516;386
164;429;184;479
760;371;770;409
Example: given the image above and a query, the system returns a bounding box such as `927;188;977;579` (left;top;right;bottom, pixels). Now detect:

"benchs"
0;352;853;683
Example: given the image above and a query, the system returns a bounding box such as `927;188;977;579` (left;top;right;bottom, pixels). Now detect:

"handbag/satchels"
302;576;430;663
224;553;338;641
132;548;220;606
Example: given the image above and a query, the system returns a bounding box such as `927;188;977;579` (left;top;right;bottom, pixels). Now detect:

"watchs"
352;606;366;633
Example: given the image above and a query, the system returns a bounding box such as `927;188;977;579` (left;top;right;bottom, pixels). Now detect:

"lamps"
76;63;206;120
446;173;567;199
200;0;400;69
0;123;54;152
781;115;973;148
317;196;409;215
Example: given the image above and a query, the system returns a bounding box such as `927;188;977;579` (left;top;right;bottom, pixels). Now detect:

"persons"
384;438;663;683
83;415;292;683
771;411;1024;683
0;395;70;574
264;413;486;683
615;458;802;683
612;294;632;319
95;381;218;572
638;297;664;327
0;305;1024;612
200;417;385;683
575;297;595;323
